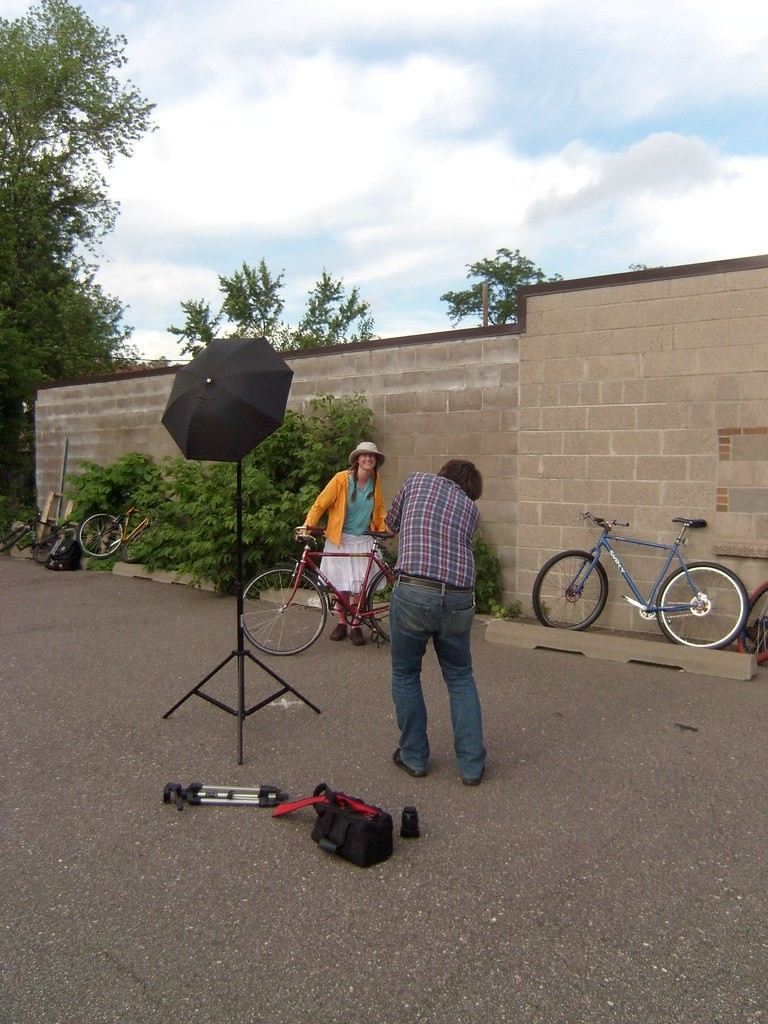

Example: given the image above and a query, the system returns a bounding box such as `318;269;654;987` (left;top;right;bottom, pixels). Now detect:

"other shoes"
350;627;366;646
330;623;347;640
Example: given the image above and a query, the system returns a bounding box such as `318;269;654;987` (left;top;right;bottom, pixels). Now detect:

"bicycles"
0;496;78;563
243;525;397;656
76;490;173;564
532;510;750;650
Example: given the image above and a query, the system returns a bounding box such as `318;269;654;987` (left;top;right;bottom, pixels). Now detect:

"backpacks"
45;539;82;571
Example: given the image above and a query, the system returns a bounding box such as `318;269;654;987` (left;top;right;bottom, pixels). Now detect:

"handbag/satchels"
310;782;394;868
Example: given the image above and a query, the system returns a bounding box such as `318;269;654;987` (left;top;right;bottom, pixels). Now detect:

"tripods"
163;458;322;766
163;782;290;811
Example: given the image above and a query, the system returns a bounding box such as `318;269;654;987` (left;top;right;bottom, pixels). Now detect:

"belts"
395;576;473;590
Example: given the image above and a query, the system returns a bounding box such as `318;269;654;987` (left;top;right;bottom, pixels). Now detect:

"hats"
349;442;385;468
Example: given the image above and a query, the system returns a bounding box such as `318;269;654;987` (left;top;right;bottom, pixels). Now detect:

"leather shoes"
392;747;428;778
463;761;485;786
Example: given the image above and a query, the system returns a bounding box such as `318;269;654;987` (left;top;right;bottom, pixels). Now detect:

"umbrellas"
161;337;294;465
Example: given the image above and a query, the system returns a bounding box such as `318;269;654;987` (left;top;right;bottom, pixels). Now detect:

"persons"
383;460;488;787
294;442;389;646
